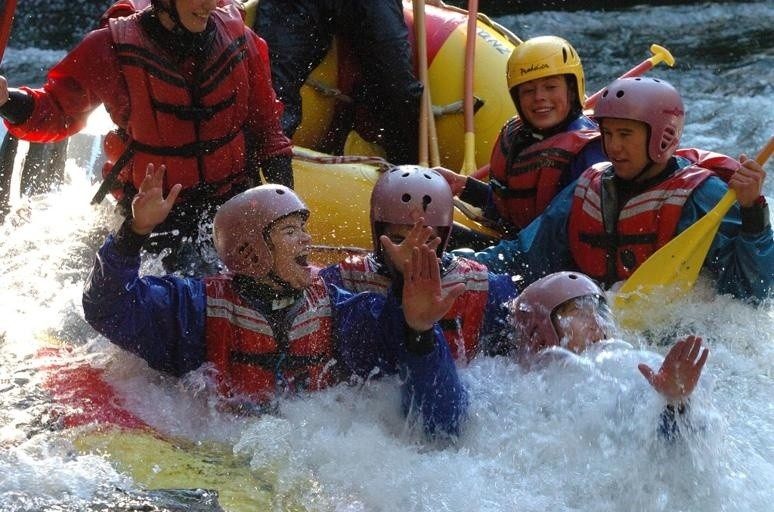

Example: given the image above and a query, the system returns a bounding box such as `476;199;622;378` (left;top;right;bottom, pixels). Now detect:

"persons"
384;243;710;440
83;161;440;416
433;35;603;235
252;1;424;168
0;1;295;271
455;76;773;305
310;164;519;359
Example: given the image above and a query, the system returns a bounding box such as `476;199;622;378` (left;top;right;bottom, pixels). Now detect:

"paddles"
412;0;478;176
614;138;774;332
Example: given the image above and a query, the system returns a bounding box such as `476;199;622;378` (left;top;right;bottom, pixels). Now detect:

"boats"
15;0;558;511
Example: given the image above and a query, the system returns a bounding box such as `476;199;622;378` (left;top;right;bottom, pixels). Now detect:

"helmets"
212;183;310;281
590;75;685;162
506;35;588;121
370;166;453;264
514;270;606;368
